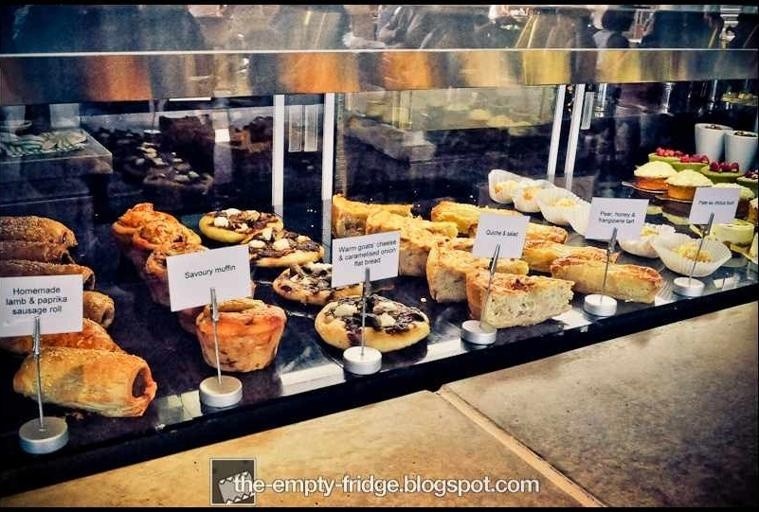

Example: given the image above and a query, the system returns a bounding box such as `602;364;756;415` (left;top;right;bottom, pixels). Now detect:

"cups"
724;130;759;174
693;123;734;165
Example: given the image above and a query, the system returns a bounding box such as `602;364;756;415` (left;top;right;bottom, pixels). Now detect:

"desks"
0;127;116;225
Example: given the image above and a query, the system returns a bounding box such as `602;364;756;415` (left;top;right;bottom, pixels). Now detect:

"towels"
0;127;89;158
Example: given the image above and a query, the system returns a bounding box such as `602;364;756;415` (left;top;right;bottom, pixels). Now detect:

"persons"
588;5;635;88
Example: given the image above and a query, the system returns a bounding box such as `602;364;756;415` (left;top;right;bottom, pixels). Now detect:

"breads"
0;148;759;419
93;84;535;209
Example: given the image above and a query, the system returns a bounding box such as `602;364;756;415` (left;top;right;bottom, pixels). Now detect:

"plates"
654;192;693;204
621;180;668;194
